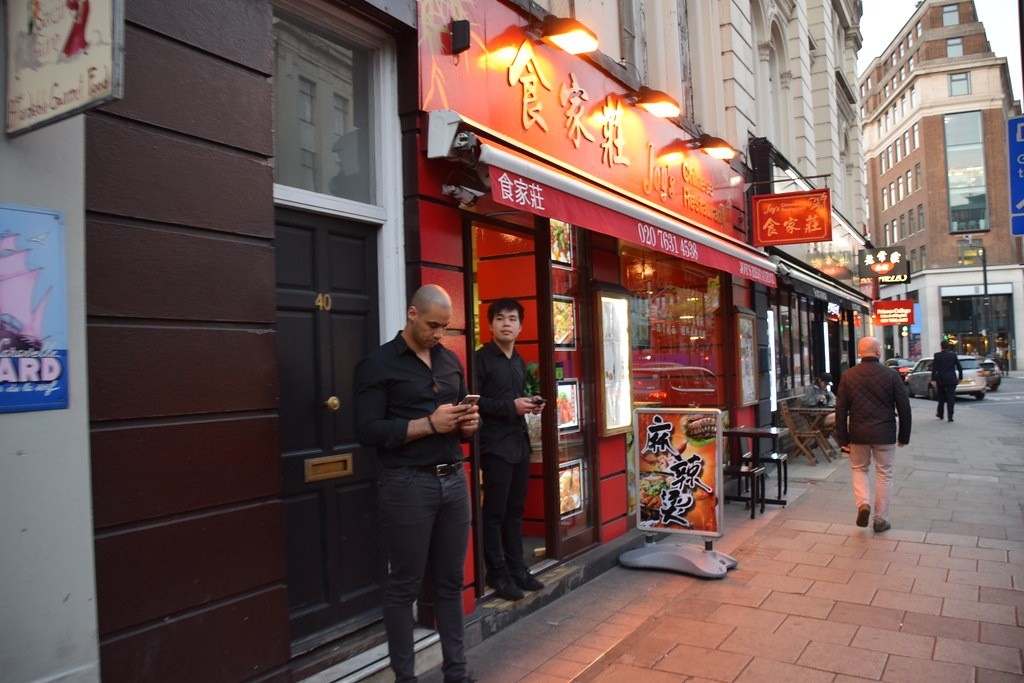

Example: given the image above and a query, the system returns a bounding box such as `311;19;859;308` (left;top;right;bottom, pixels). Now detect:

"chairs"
779;400;822;465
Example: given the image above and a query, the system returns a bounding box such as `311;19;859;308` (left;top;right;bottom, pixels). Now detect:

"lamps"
528;14;600;56
623;85;682;119
585;92;634;131
692;134;735;160
477;24;533;72
654;137;694;168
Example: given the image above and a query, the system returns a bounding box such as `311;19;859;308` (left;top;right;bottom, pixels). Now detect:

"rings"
470;420;473;423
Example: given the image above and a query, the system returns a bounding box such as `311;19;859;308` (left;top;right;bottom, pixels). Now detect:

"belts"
403;461;466;476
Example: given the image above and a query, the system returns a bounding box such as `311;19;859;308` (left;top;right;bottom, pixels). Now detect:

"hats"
819;373;834;383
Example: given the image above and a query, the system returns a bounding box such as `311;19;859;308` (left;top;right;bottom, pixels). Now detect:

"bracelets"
427;416;438;434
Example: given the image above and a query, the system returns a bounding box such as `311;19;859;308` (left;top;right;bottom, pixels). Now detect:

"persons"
993;353;1009;377
895;353;901;358
802;372;851;455
352;283;480;683
835;336;912;533
469;299;547;601
930;340;963;422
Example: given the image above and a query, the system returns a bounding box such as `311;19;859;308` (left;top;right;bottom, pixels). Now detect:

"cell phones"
533;399;548;404
460;395;481;407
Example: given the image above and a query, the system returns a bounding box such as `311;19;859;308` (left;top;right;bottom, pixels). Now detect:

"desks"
789;406;836;463
724;427;792;511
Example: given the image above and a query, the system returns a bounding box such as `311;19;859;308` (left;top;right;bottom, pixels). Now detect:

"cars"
632;366;719;409
975;356;1002;391
884;359;917;380
904;355;987;401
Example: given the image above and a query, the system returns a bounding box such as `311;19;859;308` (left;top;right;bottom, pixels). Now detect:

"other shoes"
948;419;953;422
856;504;870;526
873;520;890;533
936;414;943;419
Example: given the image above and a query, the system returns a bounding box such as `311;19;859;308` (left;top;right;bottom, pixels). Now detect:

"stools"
742;451;789;499
723;465;767;519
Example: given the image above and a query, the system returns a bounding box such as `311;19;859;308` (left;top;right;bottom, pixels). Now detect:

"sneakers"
507;563;544;591
486;565;524;601
